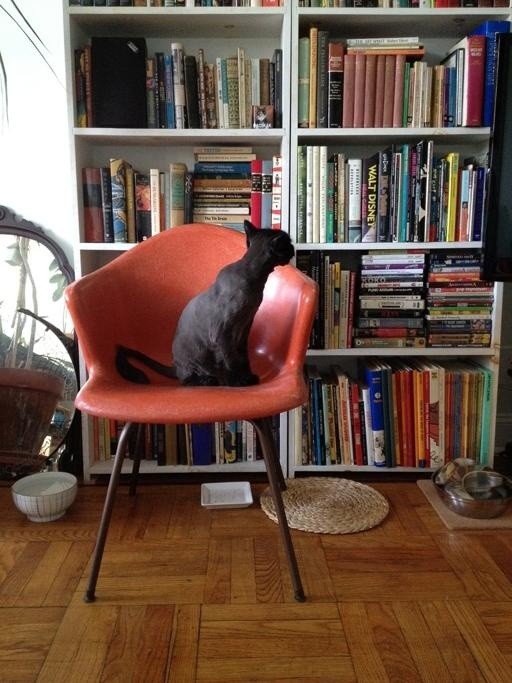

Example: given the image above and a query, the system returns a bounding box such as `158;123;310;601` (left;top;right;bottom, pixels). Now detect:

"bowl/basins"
10;472;78;524
431;463;510;520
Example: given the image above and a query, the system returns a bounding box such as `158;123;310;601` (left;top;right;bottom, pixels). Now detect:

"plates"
200;481;256;512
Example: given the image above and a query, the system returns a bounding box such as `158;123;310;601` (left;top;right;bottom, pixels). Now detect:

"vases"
0;367;64;471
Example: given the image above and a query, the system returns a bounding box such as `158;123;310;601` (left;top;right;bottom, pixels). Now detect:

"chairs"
62;220;316;607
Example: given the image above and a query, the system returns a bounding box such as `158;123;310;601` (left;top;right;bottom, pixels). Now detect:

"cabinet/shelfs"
294;0;510;480
61;0;287;479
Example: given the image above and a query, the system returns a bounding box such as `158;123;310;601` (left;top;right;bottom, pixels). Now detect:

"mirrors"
0;207;83;490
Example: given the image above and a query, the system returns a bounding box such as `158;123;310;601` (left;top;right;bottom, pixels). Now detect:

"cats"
113;219;296;388
